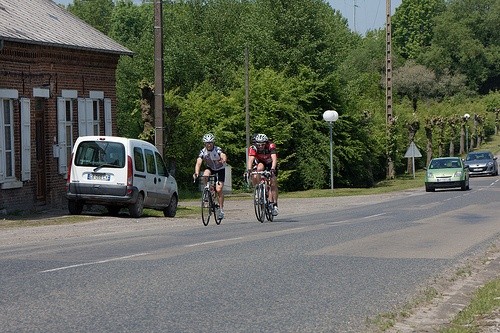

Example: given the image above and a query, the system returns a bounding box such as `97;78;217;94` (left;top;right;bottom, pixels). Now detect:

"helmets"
255;134;268;144
203;133;215;143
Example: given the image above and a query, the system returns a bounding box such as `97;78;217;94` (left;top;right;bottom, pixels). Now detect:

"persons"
243;134;278;216
193;134;227;220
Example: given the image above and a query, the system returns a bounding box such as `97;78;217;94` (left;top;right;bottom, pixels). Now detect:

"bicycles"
193;173;225;226
244;171;277;222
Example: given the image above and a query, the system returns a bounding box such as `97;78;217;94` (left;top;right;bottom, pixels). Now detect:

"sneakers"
217;209;224;220
255;188;262;204
272;206;279;216
204;196;209;202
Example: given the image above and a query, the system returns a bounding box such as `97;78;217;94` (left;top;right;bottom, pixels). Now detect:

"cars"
466;151;499;177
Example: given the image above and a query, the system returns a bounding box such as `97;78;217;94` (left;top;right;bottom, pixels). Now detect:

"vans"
66;136;179;219
424;158;471;193
